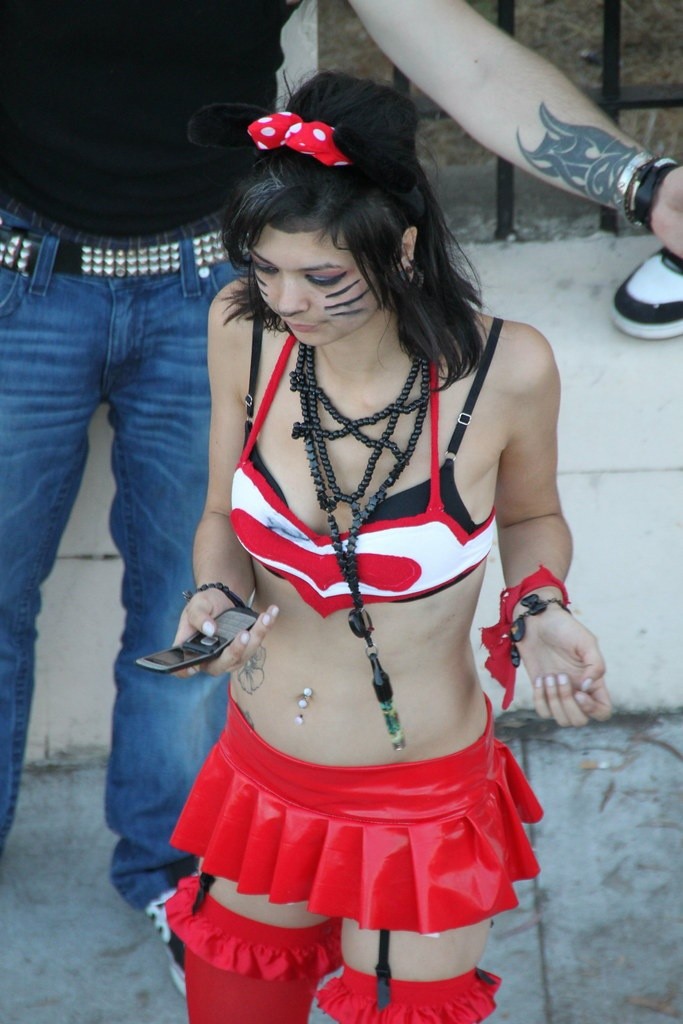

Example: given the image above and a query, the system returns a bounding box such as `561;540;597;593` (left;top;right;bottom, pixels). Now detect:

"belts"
0;226;232;276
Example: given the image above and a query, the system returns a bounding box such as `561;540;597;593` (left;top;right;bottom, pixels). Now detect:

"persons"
169;68;614;1024
0;0;683;998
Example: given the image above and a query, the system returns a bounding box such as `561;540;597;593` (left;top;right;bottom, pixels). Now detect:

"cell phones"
134;606;258;675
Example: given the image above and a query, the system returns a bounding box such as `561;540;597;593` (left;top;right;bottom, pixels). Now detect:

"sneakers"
148;887;189;993
612;247;681;338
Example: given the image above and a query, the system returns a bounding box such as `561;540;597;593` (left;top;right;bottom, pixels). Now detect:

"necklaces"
289;341;429;751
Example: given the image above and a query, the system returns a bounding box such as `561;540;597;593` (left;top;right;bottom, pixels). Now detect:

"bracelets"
480;564;572;709
613;150;679;232
181;581;246;609
